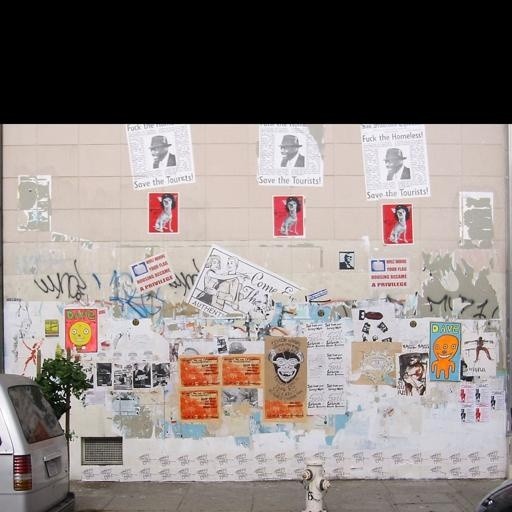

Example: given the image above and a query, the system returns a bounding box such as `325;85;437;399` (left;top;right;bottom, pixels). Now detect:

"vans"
0;374;75;512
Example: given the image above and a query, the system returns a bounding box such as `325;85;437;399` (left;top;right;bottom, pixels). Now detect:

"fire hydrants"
302;462;331;511
